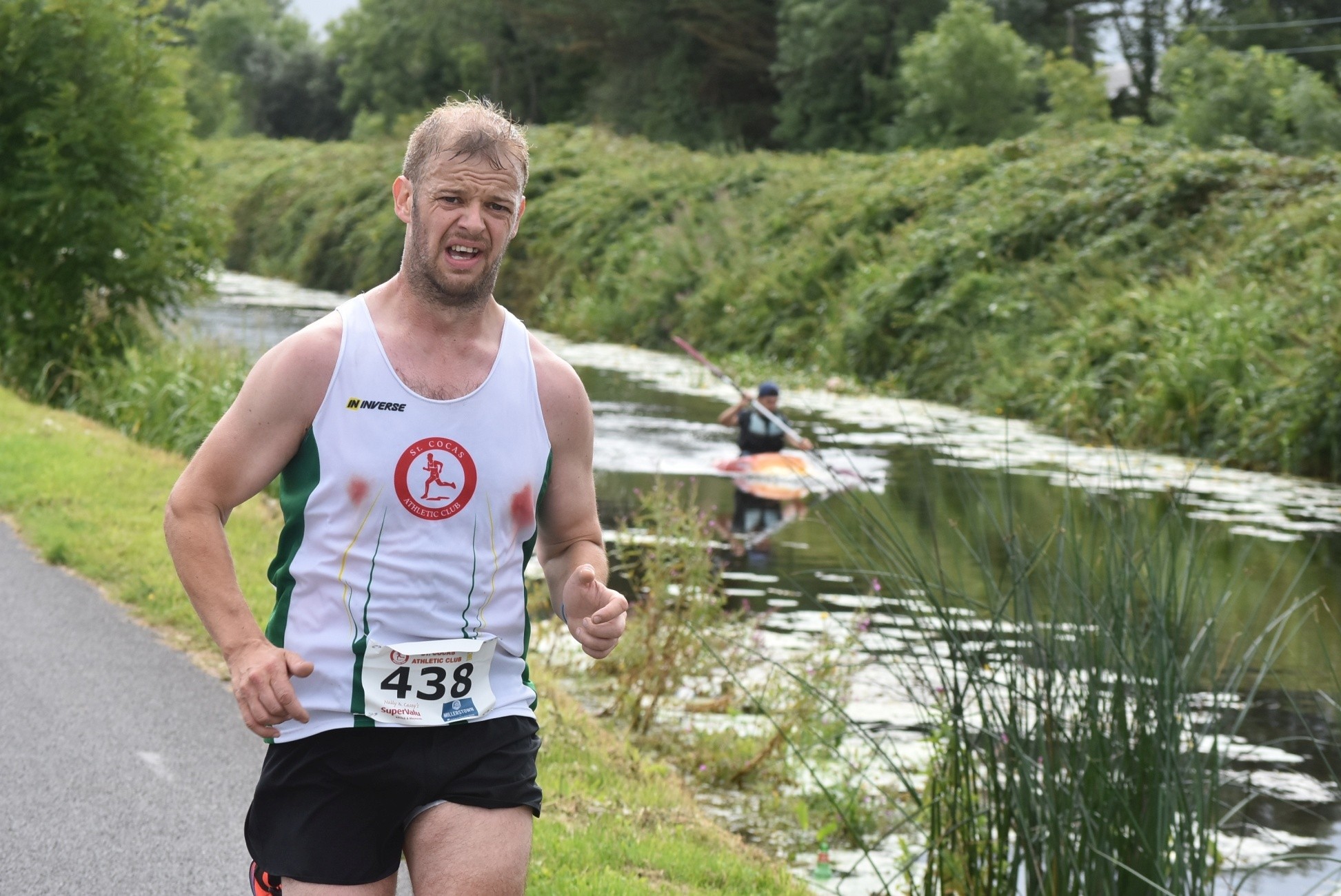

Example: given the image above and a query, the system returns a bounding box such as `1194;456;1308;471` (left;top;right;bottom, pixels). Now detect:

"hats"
756;382;779;395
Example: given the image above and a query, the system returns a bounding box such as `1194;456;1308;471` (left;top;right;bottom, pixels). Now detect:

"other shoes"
247;860;282;896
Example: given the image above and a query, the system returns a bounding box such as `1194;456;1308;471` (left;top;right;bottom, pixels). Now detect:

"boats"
720;451;809;476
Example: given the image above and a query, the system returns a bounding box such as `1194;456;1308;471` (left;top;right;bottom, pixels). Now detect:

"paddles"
672;334;836;475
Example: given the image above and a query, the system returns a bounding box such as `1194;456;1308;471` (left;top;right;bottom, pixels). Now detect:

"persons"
716;386;813;457
711;488;812;572
162;104;629;896
